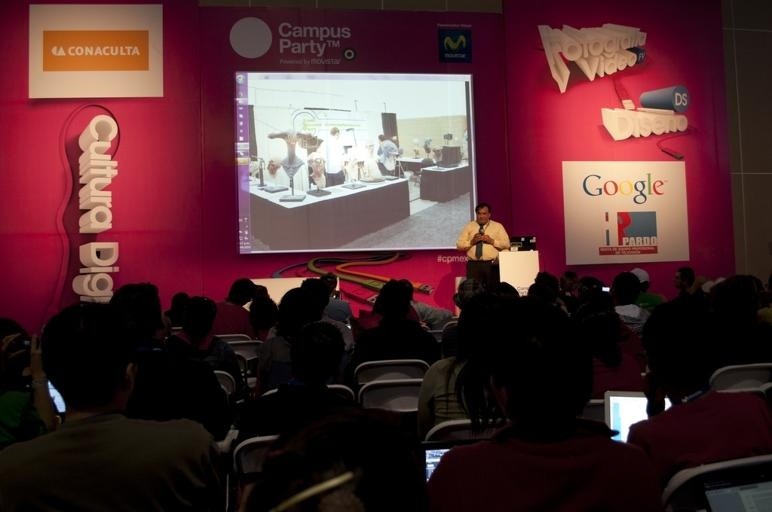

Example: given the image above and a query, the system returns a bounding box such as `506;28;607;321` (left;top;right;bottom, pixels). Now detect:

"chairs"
153;320;771;511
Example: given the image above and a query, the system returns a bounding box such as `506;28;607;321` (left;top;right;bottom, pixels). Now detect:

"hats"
630;265;651;288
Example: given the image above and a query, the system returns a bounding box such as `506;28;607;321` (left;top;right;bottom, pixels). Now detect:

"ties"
474;224;488;262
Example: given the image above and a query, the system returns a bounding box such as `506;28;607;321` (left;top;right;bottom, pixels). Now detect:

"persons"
265;125;444;194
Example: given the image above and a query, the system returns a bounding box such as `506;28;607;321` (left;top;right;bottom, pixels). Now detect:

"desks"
248;151;470;251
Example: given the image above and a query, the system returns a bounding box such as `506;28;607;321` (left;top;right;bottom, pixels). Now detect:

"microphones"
479;228;484;247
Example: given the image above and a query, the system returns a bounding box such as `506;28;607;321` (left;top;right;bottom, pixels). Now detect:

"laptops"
605;391;673;444
509;235;537;251
422;438;490;488
702;476;772;512
46;379;66;423
602;286;611;292
